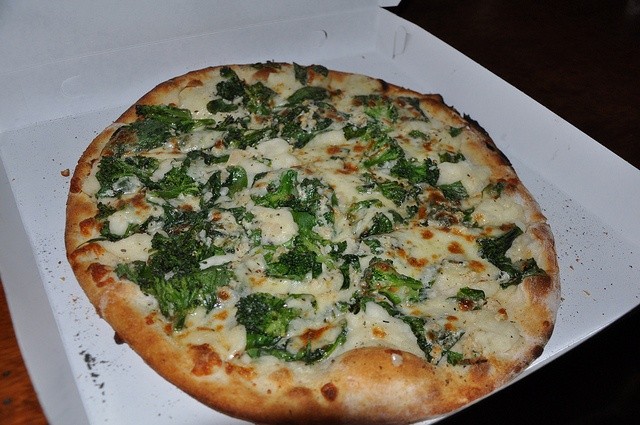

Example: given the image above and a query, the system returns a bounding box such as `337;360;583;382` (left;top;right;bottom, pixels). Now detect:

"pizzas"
65;59;559;425
